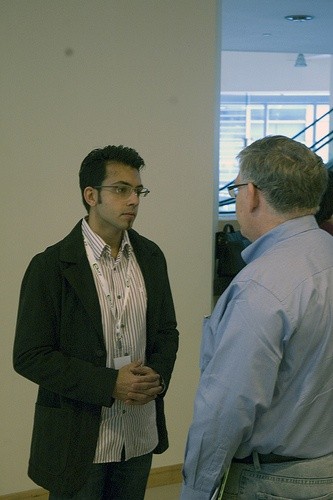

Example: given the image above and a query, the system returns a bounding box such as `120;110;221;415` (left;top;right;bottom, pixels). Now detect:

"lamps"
285;15;313;68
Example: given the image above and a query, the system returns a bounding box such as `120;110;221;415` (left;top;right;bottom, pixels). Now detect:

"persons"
12;144;178;500
181;135;333;500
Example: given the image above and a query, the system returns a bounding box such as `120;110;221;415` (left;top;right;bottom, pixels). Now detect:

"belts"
232;452;305;464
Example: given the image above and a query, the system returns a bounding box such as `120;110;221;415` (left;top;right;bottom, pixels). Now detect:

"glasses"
227;184;262;197
95;184;149;198
